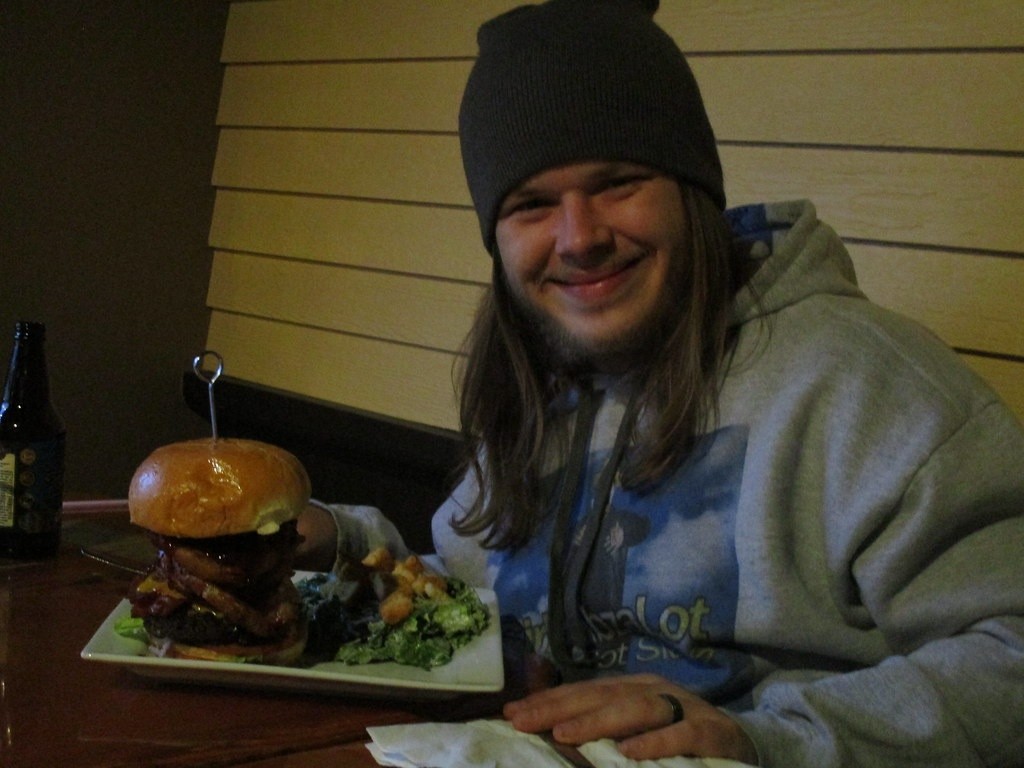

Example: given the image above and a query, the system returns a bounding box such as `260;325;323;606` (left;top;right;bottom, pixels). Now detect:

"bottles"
1;319;65;558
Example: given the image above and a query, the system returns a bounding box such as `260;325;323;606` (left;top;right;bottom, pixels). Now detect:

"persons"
150;0;1024;768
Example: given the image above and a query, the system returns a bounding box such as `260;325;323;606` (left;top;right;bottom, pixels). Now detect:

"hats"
459;1;726;266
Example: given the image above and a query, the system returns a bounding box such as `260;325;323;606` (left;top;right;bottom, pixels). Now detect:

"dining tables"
1;510;757;768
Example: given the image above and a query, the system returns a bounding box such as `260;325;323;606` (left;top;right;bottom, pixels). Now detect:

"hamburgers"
130;433;309;671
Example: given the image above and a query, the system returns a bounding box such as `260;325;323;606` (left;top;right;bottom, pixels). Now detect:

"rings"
660;690;687;726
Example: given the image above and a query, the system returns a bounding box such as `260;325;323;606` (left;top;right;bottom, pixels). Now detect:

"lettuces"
332;576;487;666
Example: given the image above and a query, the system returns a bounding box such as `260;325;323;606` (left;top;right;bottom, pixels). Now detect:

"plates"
79;569;505;704
